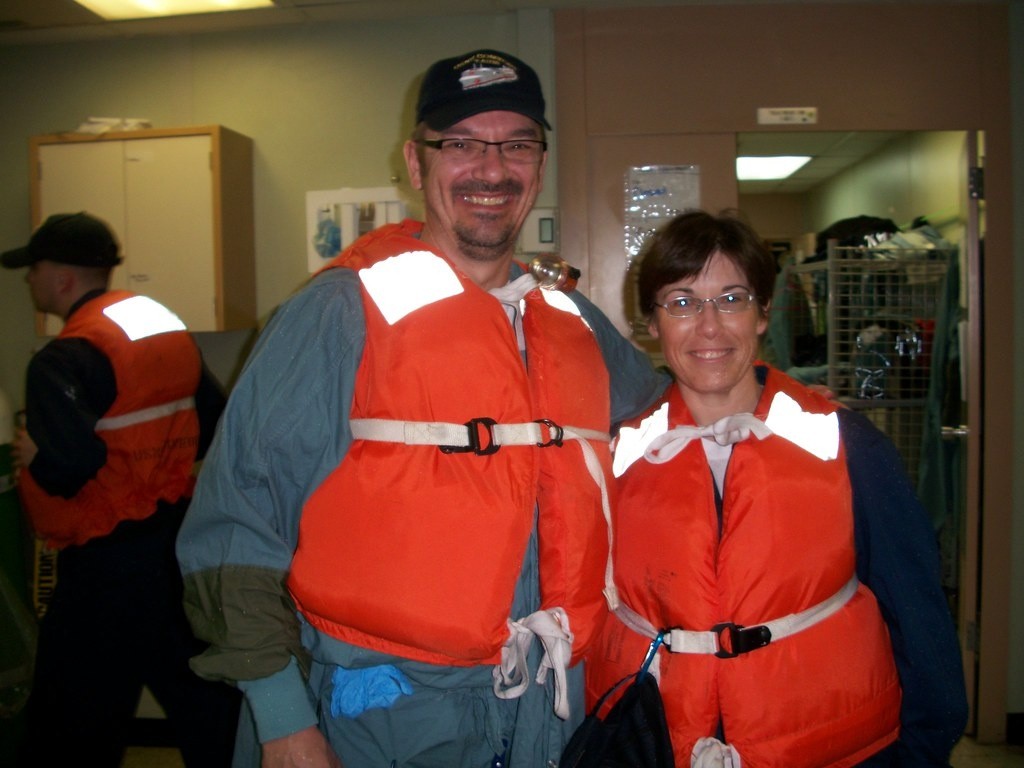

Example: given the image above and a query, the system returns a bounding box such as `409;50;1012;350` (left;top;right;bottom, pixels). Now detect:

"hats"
0;213;122;268
412;49;552;132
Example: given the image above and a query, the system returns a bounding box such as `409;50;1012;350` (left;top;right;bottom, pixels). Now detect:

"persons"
175;46;667;767
1;213;229;768
597;213;969;766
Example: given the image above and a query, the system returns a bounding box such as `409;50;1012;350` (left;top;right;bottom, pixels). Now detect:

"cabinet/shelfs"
29;127;258;338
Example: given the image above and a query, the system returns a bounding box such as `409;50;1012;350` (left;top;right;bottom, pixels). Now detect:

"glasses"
652;294;758;318
415;138;547;163
